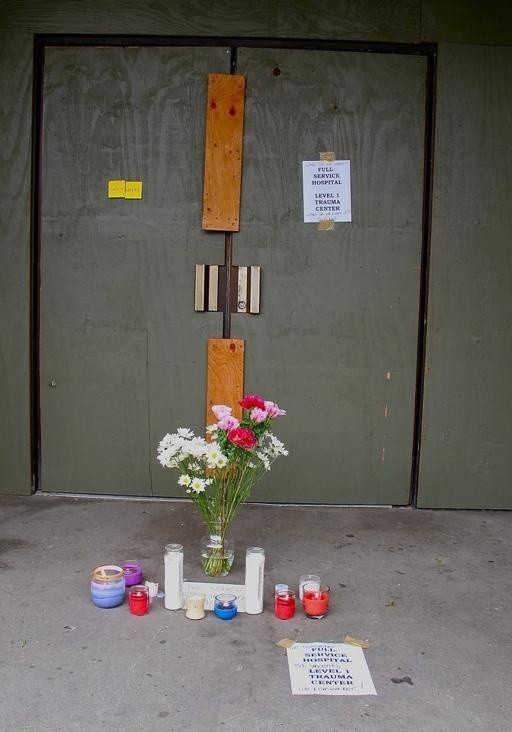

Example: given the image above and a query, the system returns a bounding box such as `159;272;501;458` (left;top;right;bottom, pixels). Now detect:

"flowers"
157;394;290;577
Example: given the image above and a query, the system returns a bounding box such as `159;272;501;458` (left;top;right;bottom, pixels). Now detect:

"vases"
201;524;234;577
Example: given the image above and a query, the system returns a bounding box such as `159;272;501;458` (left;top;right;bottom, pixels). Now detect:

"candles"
90;561;330;621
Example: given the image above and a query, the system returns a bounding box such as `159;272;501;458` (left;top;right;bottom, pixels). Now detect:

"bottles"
244;546;265;615
163;543;183;609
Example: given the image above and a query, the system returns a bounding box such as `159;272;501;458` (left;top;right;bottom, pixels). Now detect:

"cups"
129;585;149;615
275;591;295;620
214;594;236;620
274;584;288;598
186;591;204;619
299;575;321;607
90;564;125;608
118;559;142;585
302;584;330;619
